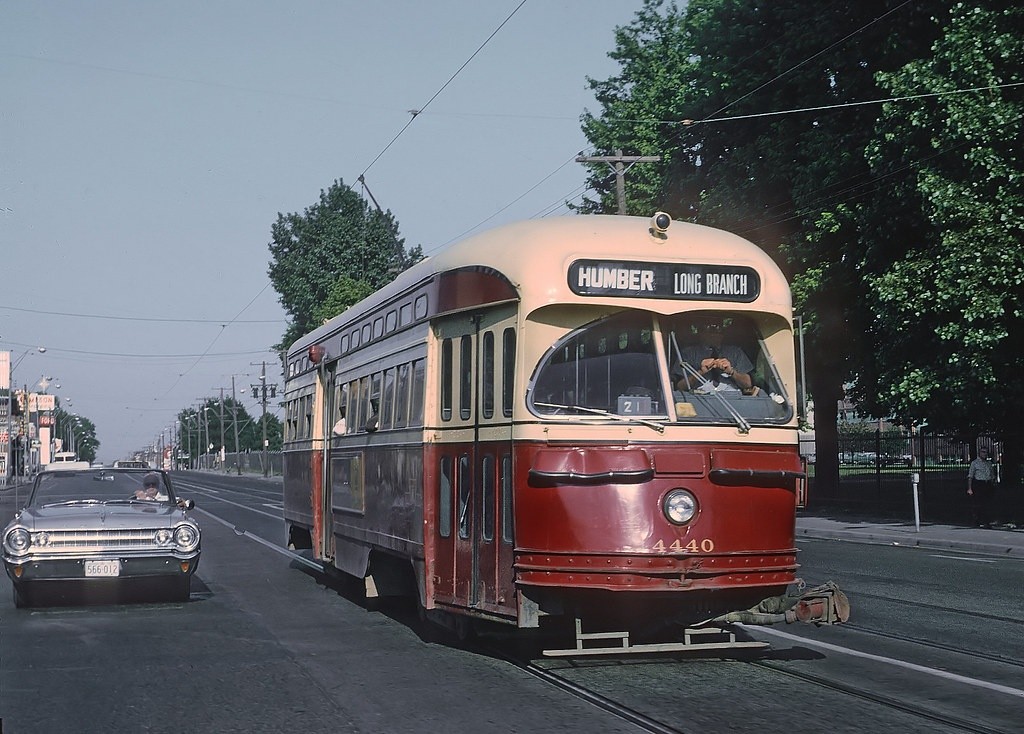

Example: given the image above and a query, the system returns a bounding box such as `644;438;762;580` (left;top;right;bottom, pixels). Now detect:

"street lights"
53;401;72;462
35;384;62;474
47;396;71;464
239;361;279;477
58;409;90;462
151;385;233;474
5;346;46;487
214;373;248;476
24;376;52;484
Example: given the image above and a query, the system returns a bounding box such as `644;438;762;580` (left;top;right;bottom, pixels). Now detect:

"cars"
799;451;1002;470
2;466;203;611
46;452;151;470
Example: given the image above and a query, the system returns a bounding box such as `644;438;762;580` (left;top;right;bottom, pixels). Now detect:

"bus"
280;207;809;666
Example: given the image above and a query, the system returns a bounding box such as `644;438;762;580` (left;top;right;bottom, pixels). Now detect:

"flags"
27;392;36;411
36;394;54;410
14;389;24;410
0;419;19;443
27;422;36;437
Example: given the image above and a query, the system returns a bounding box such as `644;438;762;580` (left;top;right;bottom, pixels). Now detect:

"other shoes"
984;523;992;529
973;522;980;529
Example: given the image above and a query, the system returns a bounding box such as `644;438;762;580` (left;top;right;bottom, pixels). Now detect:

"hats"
143;474;160;485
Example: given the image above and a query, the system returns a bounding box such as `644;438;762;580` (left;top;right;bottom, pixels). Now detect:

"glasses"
980;451;988;453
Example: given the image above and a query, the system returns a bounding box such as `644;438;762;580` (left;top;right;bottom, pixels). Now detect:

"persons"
867;452;968;467
331;415;345;434
967;446;996;527
140;507;157;512
671;321;755;394
132;473;185;507
363;412;378;431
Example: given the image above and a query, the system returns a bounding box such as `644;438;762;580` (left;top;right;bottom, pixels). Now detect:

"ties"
711;347;722;387
